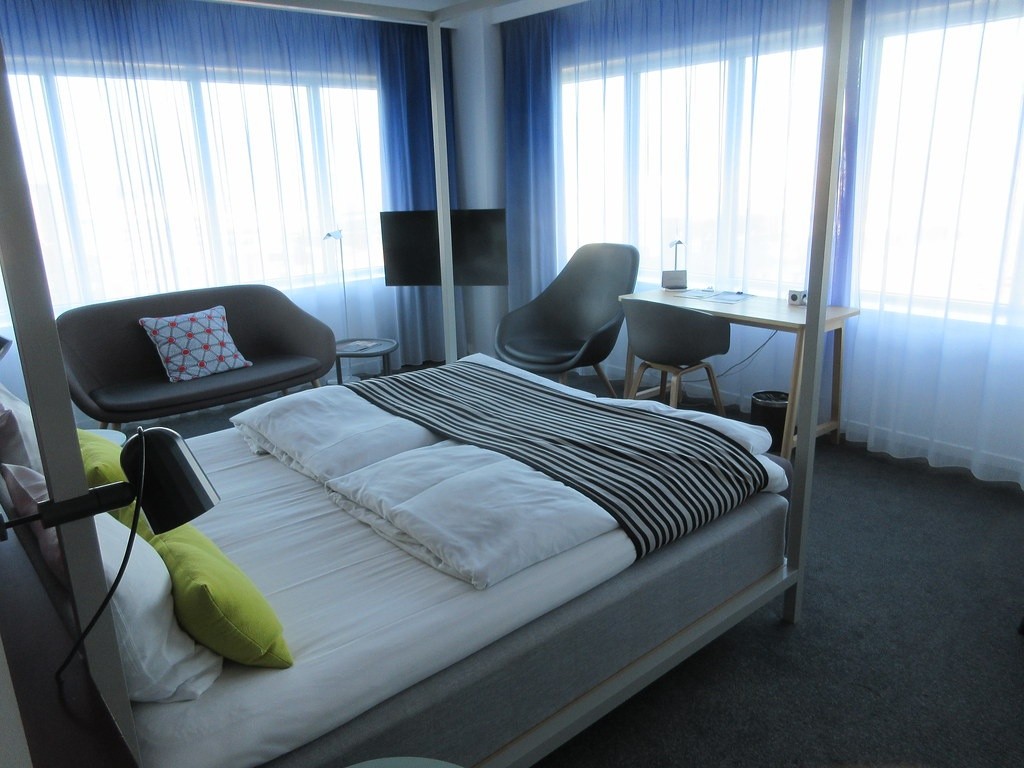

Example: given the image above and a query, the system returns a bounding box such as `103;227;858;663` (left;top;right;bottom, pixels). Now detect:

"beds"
0;0;853;768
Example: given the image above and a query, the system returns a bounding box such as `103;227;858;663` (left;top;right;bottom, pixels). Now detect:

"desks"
335;339;398;385
618;289;859;460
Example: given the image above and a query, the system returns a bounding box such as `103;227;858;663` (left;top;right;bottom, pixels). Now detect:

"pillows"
139;305;254;383
76;428;154;542
120;500;293;668
1;461;223;702
0;383;45;476
56;285;336;432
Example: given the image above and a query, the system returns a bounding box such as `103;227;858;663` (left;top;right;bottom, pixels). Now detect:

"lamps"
0;426;220;685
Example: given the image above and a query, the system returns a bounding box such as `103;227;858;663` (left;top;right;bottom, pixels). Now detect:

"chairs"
495;243;639;398
621;298;731;416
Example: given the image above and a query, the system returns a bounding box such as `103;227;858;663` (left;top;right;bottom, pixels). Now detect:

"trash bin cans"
751;391;788;456
